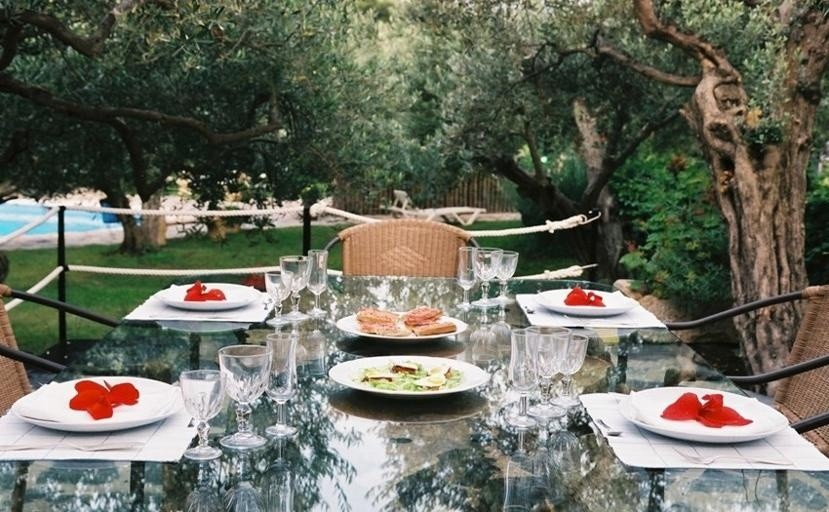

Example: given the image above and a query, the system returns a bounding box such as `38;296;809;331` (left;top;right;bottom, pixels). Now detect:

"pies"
356;306;456;337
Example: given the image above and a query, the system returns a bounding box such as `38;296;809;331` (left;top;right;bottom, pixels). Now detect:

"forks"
670;443;795;466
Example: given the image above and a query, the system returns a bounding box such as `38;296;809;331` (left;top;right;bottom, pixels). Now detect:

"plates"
329;386;490;423
666;470;777;481
9;375;186;435
161;281;262;312
336;333;469;359
327;355;494;395
563;325;639;338
618;383;790;443
157;321;250;333
337;310;470;337
538;286;639;317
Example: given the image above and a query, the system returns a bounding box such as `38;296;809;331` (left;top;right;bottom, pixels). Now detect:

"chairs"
610;285;829;512
0;282;171;510
322;219;487;299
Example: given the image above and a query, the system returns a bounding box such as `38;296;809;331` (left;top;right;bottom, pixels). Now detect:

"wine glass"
261;333;299;442
305;247;329;319
259;436;297;512
472;245;499;310
218;344;274;451
178;367;227;462
301;318;334;377
455;247;475;312
470;308;514;362
491;249;522;307
190;457;222;512
262;269;295;327
219;446;262;511
276;256;313;320
501;326;588;428
500;420;589;510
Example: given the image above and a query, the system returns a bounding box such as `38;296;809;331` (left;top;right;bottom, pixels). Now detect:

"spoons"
595;417;623;437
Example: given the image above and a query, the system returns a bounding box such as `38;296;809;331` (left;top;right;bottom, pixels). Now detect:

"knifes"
0;439;144;455
